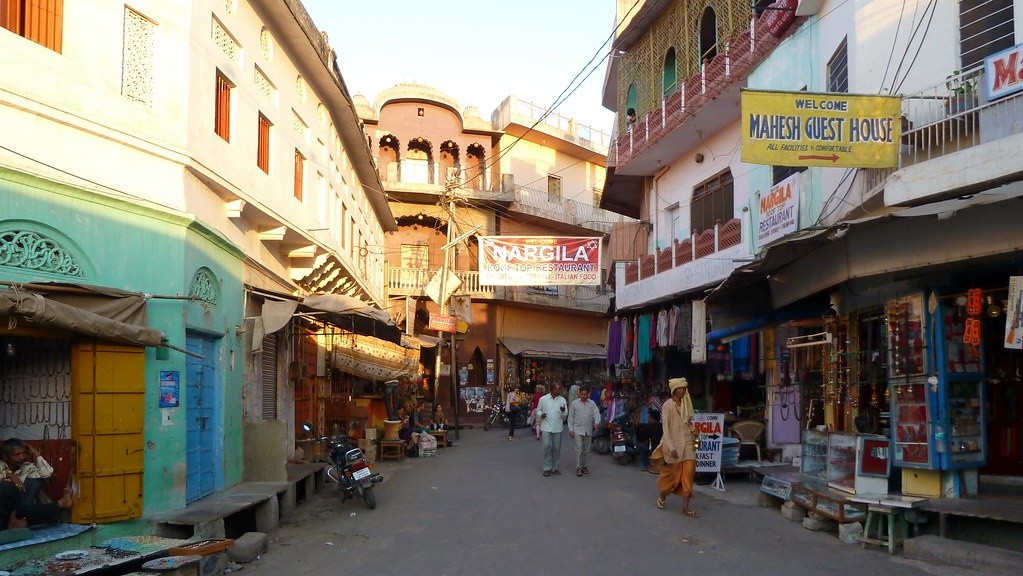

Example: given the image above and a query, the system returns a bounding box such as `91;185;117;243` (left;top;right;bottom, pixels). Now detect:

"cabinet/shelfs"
801;430;891;495
887;290;987;498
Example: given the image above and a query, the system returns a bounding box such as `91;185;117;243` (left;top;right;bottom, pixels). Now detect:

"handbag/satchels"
418;430;437;457
510;402;519;410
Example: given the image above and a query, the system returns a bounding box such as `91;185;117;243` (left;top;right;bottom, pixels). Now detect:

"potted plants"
945;70;985;115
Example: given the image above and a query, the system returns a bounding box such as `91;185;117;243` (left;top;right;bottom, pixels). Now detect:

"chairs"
728;421;765;463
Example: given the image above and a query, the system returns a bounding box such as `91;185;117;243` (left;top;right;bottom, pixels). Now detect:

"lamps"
695;153;703;163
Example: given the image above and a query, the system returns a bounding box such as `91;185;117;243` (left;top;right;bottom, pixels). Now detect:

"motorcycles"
484;398;530;430
304;421;383;509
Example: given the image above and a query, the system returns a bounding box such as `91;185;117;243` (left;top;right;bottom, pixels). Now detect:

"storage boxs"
358;428;378;464
165;539;234;576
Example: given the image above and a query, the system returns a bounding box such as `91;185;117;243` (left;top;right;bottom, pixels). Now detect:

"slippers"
688;511;700;517
657;497;665;509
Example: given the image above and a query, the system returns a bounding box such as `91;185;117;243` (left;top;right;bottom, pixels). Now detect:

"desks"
429;430;448;447
381;440;406;462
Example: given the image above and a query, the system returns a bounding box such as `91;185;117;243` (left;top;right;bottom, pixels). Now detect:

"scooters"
608;421;637;464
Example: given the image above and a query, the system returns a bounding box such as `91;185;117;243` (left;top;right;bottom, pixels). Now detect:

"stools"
764;448;782;462
860;504;909;554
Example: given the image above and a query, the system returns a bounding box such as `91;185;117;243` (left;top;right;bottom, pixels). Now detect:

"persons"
649;377;700;518
503;380;664;477
0;438;74;532
389;400;450;457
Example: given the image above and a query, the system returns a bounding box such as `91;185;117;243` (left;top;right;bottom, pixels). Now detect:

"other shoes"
543;470;550;476
551;469;561;475
577;469;582;475
640;465;650;471
537;438;540;440
582;467;589;474
508;436;520;440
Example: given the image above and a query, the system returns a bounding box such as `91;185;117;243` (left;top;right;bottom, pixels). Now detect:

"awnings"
0;277;170;350
498;336;608;363
399;332;442;351
242;284;423;353
